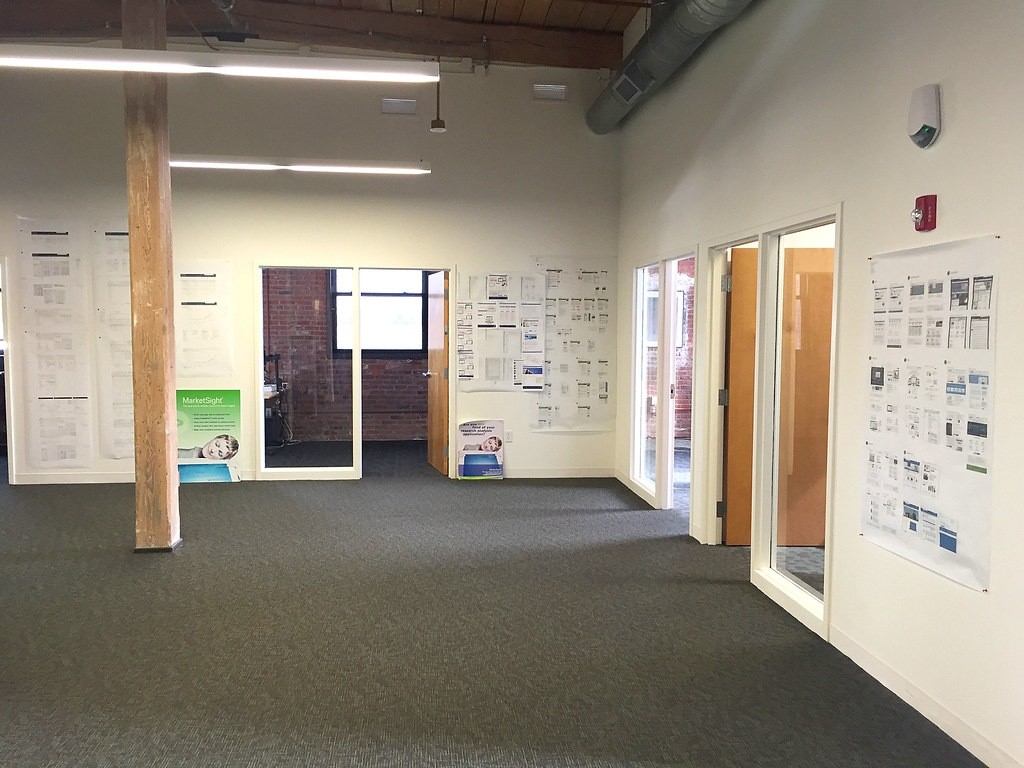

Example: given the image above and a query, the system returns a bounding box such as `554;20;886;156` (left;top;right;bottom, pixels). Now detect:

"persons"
464;436;502;453
177;434;239;461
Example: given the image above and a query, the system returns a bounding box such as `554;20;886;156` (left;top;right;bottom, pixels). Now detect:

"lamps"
168;153;432;173
0;36;441;83
